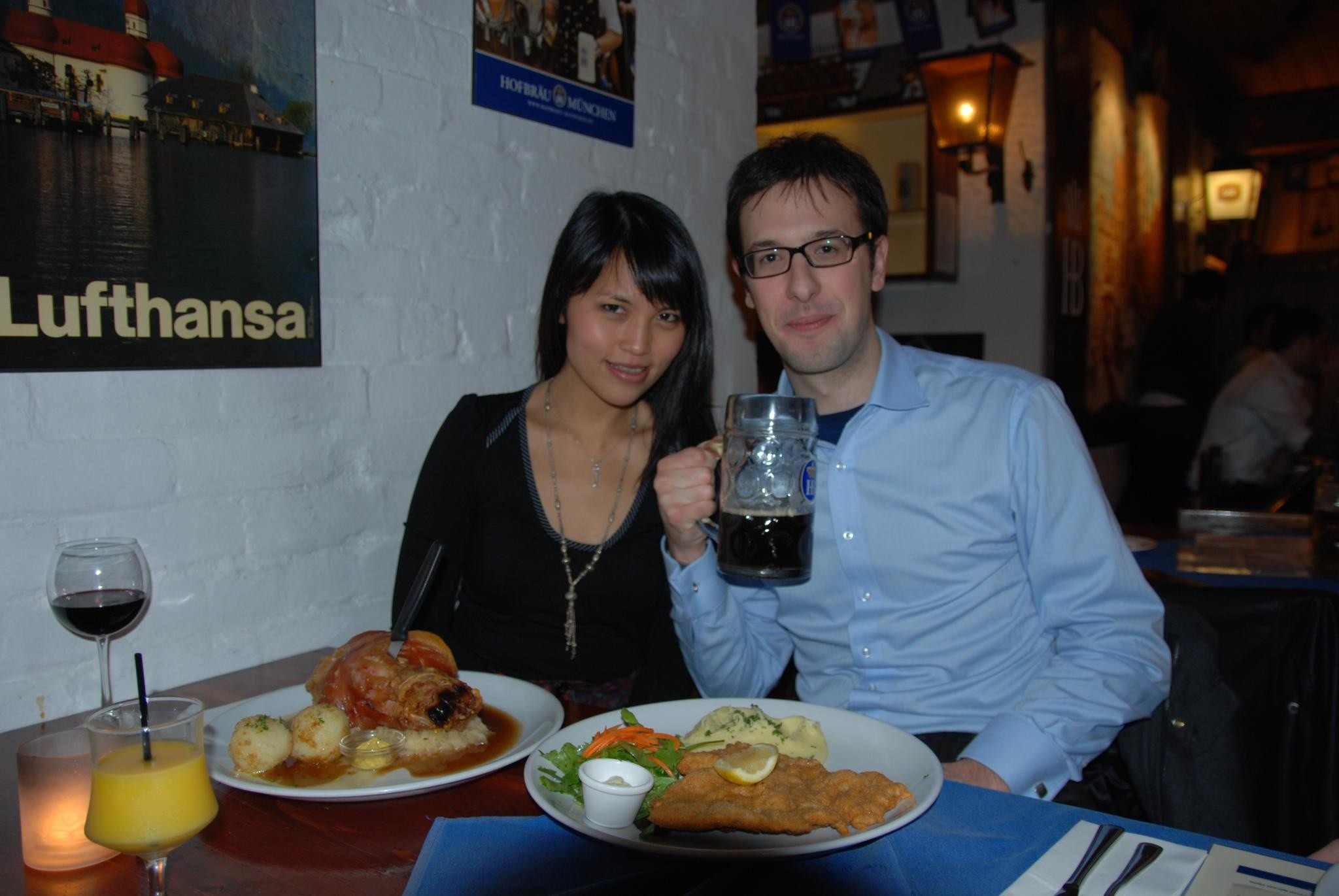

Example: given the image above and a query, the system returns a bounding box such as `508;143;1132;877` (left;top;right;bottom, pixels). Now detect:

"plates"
201;670;564;803
523;697;944;856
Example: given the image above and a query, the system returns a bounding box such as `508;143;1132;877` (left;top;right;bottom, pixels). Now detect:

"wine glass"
44;536;153;730
84;695;219;896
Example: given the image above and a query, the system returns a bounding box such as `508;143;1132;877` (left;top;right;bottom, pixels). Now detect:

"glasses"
741;229;872;279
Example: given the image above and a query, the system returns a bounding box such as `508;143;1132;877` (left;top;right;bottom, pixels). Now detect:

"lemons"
713;743;780;786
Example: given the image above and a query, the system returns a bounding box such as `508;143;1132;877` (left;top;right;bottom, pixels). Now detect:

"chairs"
1049;589;1339;858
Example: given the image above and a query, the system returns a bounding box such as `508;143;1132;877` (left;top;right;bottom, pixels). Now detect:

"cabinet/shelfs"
756;44;959;281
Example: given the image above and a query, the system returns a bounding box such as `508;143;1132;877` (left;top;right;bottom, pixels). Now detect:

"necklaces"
540;371;638;660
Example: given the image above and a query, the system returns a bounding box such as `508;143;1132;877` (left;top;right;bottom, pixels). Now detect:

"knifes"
387;539;445;660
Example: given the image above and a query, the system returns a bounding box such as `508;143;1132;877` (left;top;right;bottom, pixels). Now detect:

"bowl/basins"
338;730;407;770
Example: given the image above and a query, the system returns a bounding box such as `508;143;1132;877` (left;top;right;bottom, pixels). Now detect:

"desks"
1118;524;1339;595
0;647;1339;896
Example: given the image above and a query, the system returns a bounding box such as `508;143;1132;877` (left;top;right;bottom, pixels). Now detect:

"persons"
533;0;635;101
1189;305;1322;516
652;131;1173;800
388;190;723;731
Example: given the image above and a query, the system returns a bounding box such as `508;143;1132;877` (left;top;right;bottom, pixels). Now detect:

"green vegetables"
538;706;727;822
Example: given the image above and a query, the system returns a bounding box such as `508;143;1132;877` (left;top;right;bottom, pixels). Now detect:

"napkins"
1000;819;1208;896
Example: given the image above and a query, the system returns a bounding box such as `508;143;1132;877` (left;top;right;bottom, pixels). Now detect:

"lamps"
1197;141;1263;261
919;42;1035;204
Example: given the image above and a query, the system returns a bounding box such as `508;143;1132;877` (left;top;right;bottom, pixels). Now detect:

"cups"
578;757;654;829
696;393;816;588
